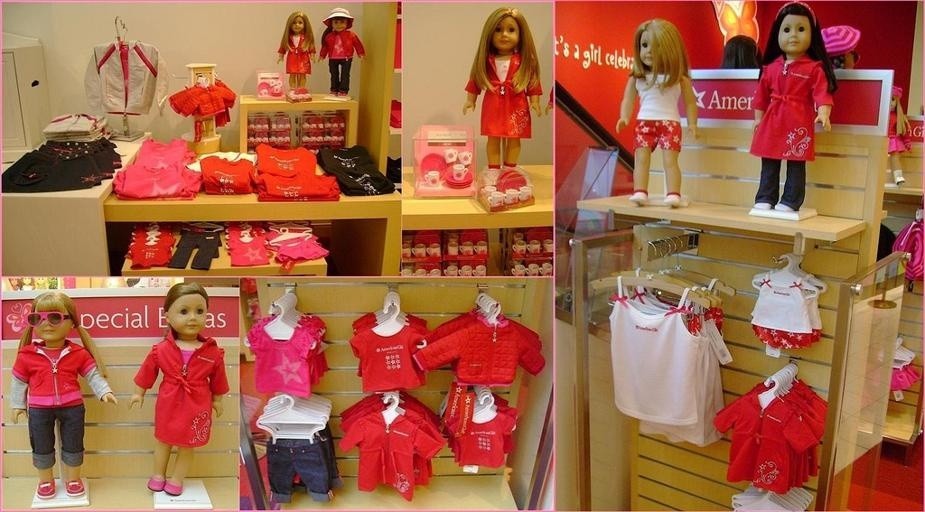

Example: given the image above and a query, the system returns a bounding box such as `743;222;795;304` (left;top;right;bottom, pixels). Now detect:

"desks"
120;228;328;277
401;164;553;276
0;130;150;278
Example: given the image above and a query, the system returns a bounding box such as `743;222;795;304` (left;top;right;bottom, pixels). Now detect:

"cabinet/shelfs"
0;33;54;161
238;95;358;155
568;126;889;512
104;147;401;277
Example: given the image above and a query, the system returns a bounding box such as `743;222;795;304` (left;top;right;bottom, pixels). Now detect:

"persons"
720;34;762;69
749;0;837;211
318;9;366;96
10;292;119;499
462;6;543;170
822;26;862;69
887;84;912;187
615;17;700;208
127;279;229;495
276;10;317;90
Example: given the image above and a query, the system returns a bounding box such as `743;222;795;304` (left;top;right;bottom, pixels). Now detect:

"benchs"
0;347;239;511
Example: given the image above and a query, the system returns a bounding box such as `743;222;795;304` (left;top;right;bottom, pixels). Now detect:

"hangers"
463;385;501;423
591;235;736;312
889;338;915;373
749;253;829;301
256;291;325;350
730;481;814;512
756;363;800;409
113;15;129;44
255;392;333;446
368;293;425;348
468;291;510;327
377;391;409;429
